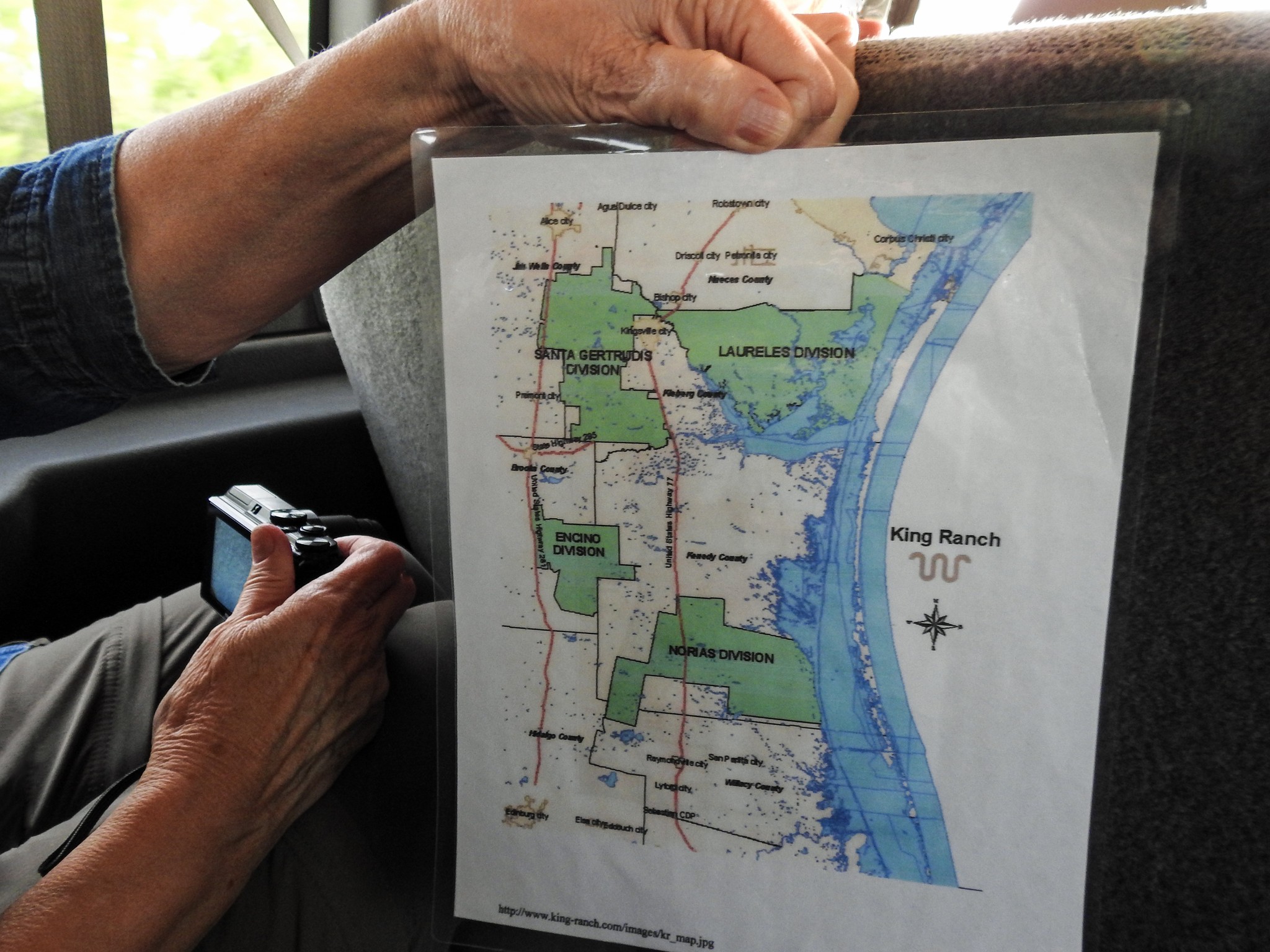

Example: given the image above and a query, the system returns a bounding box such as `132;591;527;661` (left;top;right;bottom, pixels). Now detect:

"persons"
0;0;859;952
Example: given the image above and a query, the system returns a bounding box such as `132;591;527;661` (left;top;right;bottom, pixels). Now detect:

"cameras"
199;484;341;623
